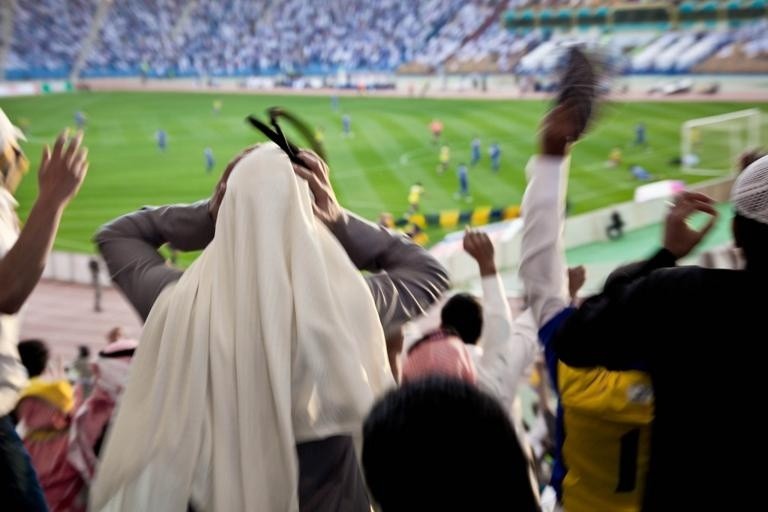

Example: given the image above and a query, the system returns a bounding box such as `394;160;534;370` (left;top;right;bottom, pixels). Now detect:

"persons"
0;1;767;512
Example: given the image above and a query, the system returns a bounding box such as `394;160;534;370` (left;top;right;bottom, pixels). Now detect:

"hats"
733;155;768;225
